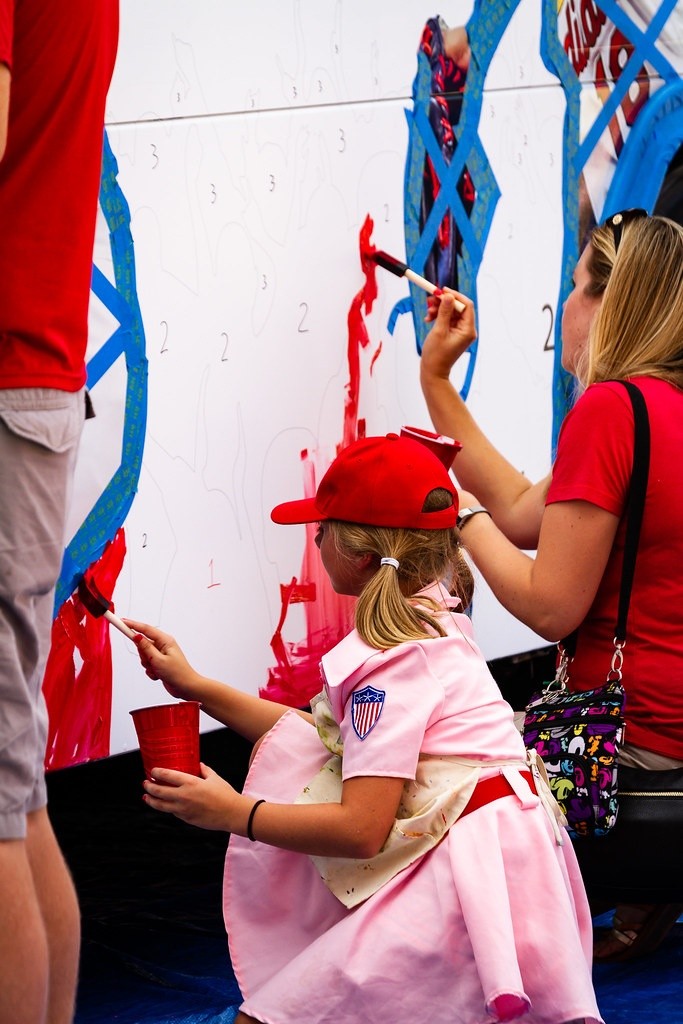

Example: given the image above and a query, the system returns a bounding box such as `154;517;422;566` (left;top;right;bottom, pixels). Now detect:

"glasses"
606;208;646;253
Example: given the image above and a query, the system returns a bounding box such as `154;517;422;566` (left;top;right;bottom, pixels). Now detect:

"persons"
0;0;124;1023
119;431;607;1024
422;209;683;961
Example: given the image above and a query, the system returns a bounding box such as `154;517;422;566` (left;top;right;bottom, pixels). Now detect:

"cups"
400;426;460;473
129;701;202;788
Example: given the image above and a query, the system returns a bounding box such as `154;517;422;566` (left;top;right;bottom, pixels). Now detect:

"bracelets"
456;505;491;530
248;800;265;841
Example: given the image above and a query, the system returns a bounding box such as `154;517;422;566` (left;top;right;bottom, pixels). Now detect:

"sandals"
594;903;683;961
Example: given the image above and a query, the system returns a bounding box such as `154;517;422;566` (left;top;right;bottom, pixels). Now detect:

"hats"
270;433;459;530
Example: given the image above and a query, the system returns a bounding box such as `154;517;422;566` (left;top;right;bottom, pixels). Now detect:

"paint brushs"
73;577;138;640
362;242;465;312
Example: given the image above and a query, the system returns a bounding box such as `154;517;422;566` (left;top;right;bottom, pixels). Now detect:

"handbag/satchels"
522;682;622;843
